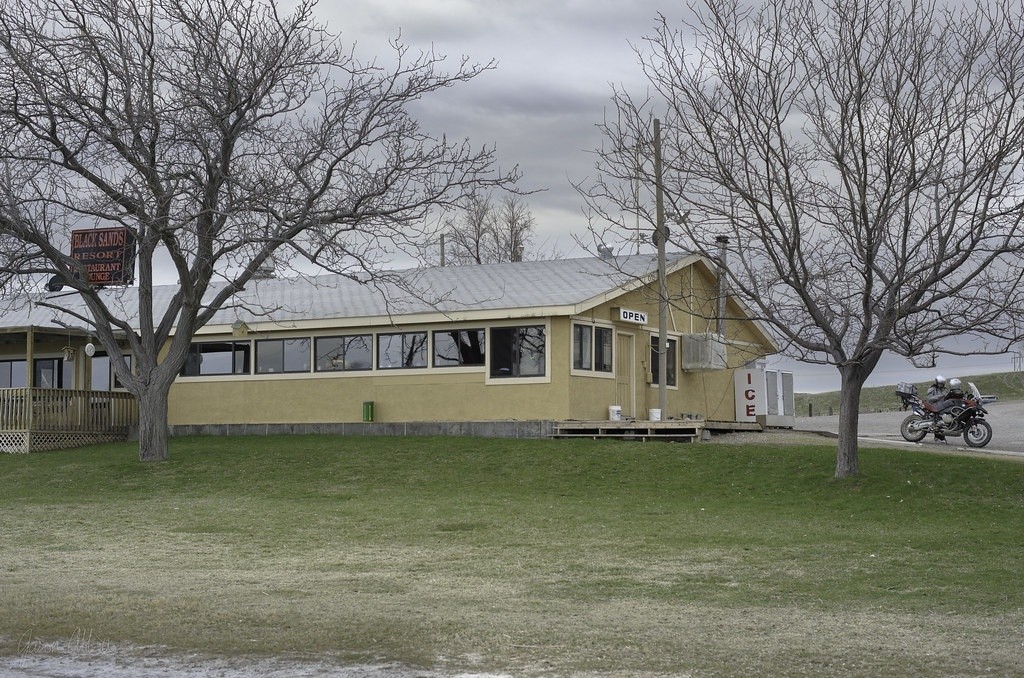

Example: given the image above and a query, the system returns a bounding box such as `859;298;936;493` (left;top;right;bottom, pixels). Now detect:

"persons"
926;375;949;444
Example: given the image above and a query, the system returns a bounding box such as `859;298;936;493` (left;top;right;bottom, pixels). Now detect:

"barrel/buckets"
608;406;622;421
648;409;662;421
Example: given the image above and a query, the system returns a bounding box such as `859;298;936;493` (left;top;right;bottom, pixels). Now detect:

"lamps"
61;330;76;362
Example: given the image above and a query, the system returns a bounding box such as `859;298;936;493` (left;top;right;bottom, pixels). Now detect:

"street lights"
658;209;691;420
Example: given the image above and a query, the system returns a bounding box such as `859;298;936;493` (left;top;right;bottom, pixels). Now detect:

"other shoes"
934;437;947;445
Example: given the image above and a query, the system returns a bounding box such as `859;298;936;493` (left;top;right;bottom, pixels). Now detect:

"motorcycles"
896;382;993;448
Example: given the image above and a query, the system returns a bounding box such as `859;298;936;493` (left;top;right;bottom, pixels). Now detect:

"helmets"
935;375;947;386
950;378;962;388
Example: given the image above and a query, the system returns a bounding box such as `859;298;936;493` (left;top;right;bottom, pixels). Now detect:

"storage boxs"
895;383;918;397
